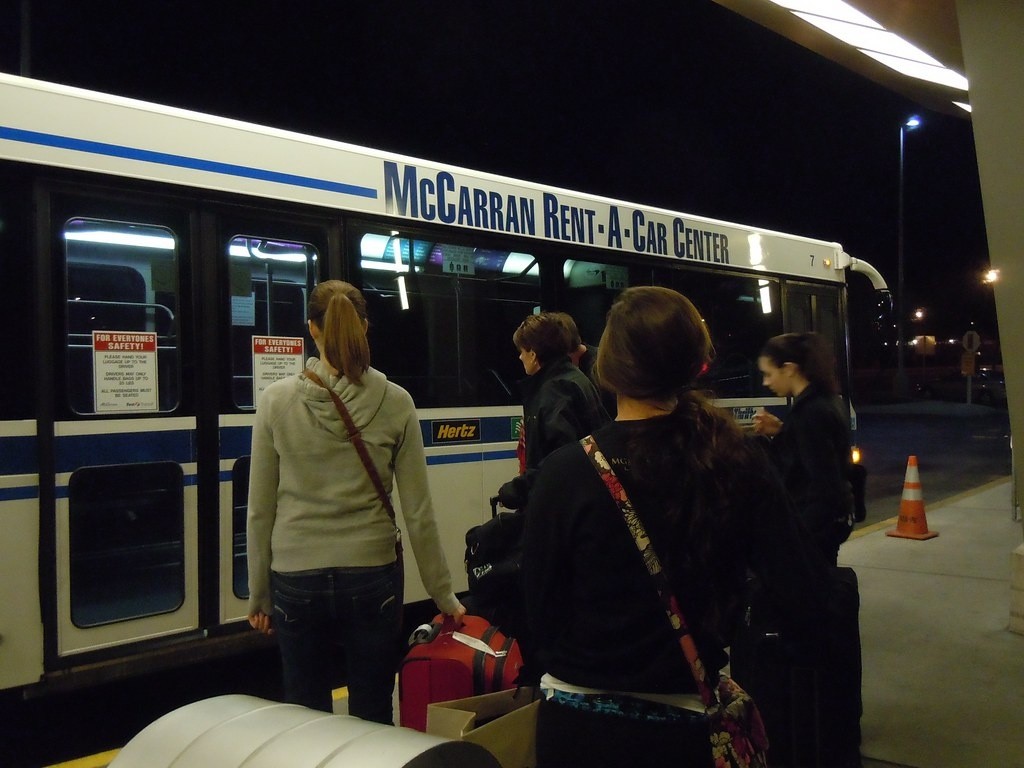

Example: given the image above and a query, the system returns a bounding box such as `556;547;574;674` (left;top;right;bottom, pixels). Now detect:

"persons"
751;331;853;574
244;280;467;727
512;308;617;473
495;284;751;768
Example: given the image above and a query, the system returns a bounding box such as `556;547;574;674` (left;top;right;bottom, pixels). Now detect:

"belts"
274;562;396;577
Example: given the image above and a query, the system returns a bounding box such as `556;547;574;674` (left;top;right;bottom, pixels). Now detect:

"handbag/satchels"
394;540;404;634
845;463;866;523
704;675;770;768
426;641;541;768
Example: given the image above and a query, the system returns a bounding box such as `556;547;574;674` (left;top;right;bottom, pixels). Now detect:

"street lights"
898;115;922;380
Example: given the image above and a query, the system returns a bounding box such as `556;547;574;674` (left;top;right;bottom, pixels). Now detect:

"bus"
1;74;904;768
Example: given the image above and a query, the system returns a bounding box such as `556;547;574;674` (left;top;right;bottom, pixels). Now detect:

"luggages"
466;498;531;661
731;565;863;768
398;612;524;733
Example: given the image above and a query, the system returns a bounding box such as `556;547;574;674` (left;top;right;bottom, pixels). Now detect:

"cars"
939;364;1006;407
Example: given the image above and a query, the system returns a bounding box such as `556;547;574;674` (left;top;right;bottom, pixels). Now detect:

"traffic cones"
885;456;939;541
